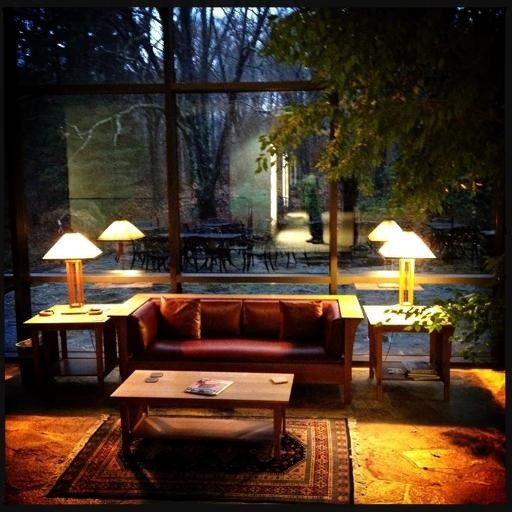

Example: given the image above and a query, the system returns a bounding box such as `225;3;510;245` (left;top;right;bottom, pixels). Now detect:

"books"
400;359;441;381
183;376;237;397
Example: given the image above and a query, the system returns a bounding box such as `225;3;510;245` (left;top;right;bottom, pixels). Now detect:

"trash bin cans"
15;338;43;394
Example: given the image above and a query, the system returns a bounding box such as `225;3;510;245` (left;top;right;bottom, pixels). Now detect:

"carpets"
47;413;357;505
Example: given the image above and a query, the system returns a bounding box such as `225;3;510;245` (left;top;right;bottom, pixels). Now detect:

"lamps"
377;229;437;316
98;218;150;284
43;228;103;311
366;218;405;288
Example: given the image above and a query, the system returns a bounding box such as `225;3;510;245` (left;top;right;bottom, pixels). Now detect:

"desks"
354;270;426;292
361;302;457;405
90;270;155;292
25;302;120;398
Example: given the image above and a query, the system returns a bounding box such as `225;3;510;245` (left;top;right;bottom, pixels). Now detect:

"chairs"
130;215;311;280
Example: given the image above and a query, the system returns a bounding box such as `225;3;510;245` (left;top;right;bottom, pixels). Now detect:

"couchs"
111;291;365;410
